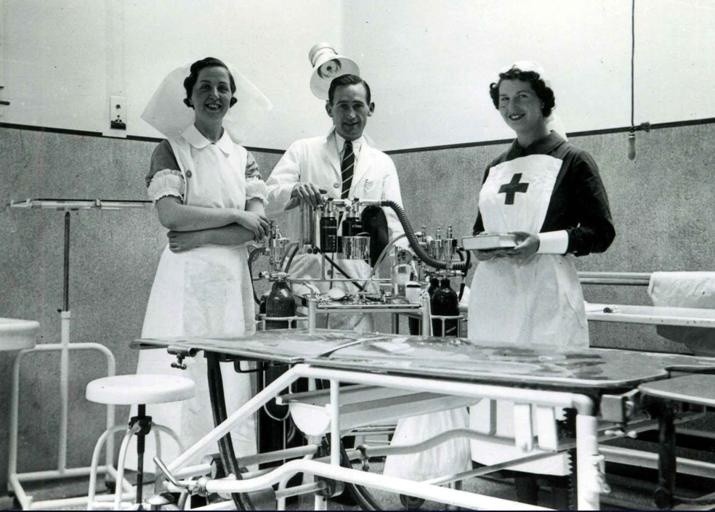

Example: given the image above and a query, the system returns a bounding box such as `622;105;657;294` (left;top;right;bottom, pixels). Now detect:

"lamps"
304;43;362;101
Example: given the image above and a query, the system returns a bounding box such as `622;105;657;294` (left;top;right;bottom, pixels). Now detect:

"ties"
338;141;354;227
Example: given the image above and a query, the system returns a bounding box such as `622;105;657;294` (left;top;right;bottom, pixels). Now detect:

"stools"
84;374;199;510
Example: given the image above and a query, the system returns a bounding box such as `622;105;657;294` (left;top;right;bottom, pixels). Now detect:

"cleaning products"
428;278;460;337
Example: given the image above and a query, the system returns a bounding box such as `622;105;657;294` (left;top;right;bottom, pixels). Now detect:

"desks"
130;327;714;509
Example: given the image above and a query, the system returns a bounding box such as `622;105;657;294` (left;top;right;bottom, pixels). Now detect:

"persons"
261;72;407;509
464;64;619;512
115;55;273;477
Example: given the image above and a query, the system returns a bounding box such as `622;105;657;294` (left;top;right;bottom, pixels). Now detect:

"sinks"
1;317;40;351
